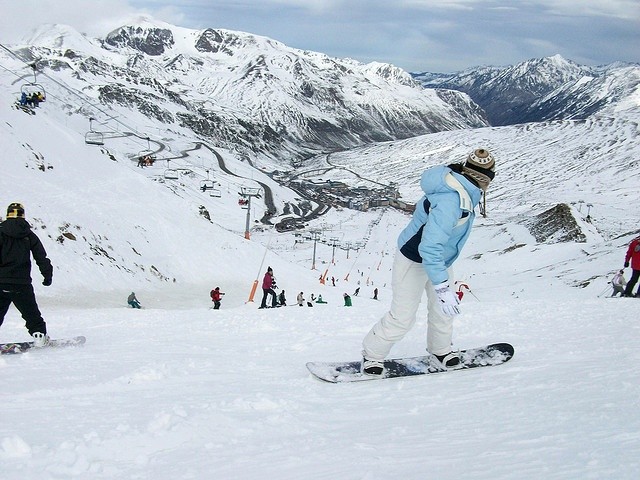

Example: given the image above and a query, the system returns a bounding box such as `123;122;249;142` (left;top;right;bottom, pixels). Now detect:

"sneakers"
364;358;383;374
33;331;48;347
437;353;459;366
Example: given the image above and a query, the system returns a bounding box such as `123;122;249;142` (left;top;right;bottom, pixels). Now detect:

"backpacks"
210;290;217;297
612;276;620;285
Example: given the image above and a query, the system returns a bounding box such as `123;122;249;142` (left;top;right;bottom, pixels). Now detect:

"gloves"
40;271;52;286
433;282;461;317
624;262;629;267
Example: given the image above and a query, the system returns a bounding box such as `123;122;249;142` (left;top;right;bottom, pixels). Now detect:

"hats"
215;287;219;290
268;266;272;271
465;149;495;180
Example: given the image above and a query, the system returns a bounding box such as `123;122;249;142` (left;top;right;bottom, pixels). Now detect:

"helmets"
6;203;25;218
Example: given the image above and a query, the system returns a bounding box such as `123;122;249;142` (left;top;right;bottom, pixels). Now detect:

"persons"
361;149;496;376
296;291;304;307
127;291;141;309
454;280;470;301
624;236;639;296
308;266;379;306
261;266;276;308
18;92;44;109
212;287;222;309
612;270;627;296
0;202;53;349
279;290;286;306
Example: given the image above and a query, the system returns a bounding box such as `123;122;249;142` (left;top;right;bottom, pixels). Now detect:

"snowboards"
0;336;87;356
306;343;514;383
